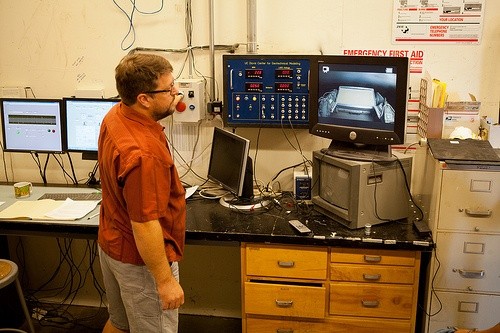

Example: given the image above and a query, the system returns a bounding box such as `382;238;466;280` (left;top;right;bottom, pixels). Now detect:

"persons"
92;50;189;333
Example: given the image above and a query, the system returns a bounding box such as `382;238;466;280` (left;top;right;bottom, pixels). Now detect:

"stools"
0;258;36;333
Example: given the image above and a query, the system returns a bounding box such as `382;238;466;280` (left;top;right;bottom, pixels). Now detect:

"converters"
413;220;432;237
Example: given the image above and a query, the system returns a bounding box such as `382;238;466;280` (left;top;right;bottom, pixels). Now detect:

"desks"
0;184;435;253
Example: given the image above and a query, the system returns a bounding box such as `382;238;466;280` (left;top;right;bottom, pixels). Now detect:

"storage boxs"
418;79;444;139
442;101;482;138
481;115;500;149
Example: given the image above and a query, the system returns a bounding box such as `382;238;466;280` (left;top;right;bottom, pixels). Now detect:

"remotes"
288;219;312;235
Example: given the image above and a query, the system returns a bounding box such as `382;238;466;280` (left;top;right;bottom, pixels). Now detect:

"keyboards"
36;192;102;205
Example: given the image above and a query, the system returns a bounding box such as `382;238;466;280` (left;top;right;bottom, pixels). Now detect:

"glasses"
143;80;175;96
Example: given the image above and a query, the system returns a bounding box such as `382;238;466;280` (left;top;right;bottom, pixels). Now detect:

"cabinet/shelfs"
411;143;500;333
240;241;421;332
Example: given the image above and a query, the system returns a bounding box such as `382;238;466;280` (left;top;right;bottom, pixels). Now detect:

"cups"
13;182;33;198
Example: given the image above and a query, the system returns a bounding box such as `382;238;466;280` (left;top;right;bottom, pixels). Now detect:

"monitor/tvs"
307;54;409;162
205;126;261;207
0;98;64;154
61;97;122;161
221;54;310;128
